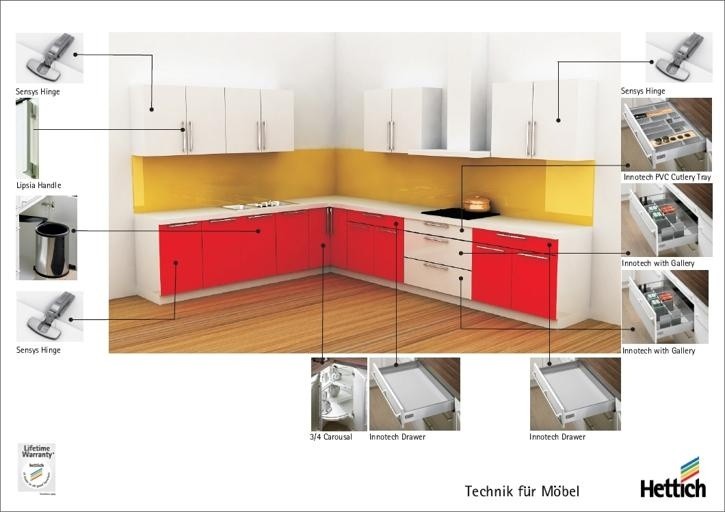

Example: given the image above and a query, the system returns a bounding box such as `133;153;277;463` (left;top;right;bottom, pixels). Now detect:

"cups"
329;384;341;398
19;214;71;279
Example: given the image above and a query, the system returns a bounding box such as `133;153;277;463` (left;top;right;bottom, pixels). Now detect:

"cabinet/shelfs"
199;212;277;299
274;206;331;283
488;77;599;163
329;203;403;292
129;78;227;159
400;217;472;315
132;218;199;306
360;86;444;157
224;84;298;156
471;225;595;332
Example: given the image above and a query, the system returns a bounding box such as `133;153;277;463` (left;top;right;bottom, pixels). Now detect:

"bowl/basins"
463;195;491;212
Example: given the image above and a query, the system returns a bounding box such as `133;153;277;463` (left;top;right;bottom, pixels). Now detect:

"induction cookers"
421;207;500;221
